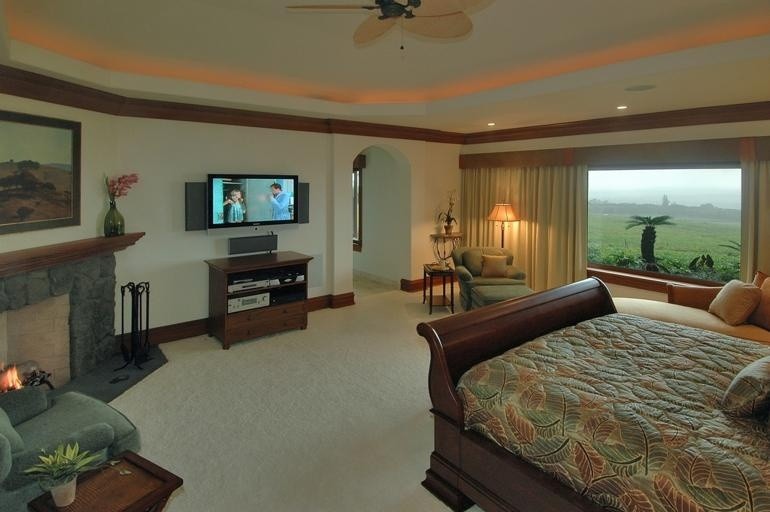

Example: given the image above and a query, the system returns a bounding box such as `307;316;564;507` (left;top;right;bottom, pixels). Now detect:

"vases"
103;201;125;238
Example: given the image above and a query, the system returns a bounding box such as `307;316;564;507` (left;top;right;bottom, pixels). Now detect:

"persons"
268;183;290;220
223;188;246;224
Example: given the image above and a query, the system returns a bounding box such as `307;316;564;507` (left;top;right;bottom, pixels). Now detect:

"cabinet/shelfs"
204;250;314;350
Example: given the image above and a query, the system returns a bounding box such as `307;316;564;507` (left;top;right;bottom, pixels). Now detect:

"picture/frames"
0;109;81;236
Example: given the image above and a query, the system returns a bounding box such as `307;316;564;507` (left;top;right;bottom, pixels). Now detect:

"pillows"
752;271;770;288
481;255;507;279
708;279;763;326
747;276;770;332
720;355;770;419
462;250;481;276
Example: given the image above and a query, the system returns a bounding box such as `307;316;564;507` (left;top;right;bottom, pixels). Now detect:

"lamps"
487;204;520;248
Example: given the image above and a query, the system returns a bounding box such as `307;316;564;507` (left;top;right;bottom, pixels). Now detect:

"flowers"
17;439;133;494
105;170;141;201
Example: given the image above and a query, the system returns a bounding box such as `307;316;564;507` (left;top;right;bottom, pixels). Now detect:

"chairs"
0;386;142;512
450;247;526;311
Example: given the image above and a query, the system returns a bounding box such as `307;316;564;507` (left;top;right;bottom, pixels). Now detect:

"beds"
611;281;770;343
416;275;770;511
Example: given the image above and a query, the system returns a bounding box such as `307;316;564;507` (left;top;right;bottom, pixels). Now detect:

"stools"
423;264;455;315
471;284;533;308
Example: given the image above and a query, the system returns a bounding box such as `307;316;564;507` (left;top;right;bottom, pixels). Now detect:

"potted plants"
437;190;457;235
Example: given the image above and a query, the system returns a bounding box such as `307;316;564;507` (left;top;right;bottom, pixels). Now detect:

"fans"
285;0;474;50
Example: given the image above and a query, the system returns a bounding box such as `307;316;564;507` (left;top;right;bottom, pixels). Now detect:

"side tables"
27;448;184;512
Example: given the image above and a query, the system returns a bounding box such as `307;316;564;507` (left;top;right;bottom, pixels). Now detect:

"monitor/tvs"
208;173;299;230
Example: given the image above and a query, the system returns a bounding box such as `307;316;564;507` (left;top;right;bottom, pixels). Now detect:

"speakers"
298;183;310;223
185;181;207;231
227;234;278;255
